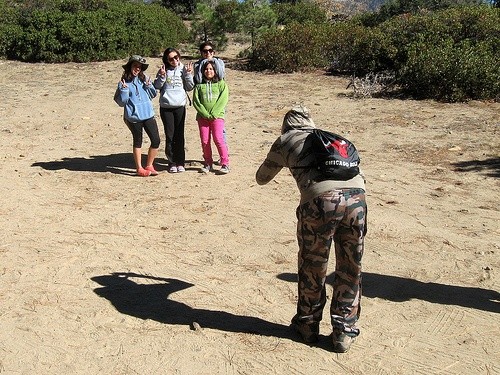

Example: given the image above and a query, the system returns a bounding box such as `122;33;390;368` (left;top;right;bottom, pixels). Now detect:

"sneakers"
219;166;230;174
200;163;212;173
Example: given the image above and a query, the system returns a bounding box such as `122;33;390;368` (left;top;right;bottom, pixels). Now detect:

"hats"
122;55;149;72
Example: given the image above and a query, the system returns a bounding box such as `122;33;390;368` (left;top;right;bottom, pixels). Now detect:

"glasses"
167;54;179;62
202;49;213;54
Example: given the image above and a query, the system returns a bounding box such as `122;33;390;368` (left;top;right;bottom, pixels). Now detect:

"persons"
193;41;224;84
113;55;160;177
193;60;230;174
154;46;195;173
256;105;367;353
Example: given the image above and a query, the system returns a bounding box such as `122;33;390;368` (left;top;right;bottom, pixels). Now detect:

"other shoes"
178;165;185;171
333;333;350;353
170;166;177;173
137;168;151;176
145;165;159;175
290;321;319;342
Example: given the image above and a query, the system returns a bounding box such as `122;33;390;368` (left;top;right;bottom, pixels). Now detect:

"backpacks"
290;127;360;181
